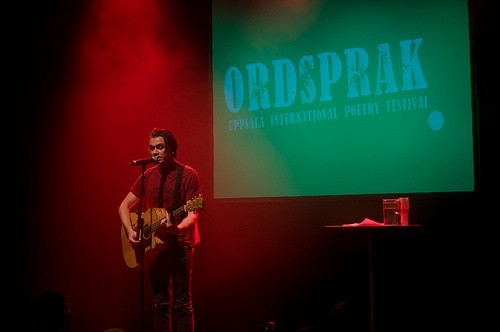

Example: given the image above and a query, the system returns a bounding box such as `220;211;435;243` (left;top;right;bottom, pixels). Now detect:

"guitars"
119;194;204;271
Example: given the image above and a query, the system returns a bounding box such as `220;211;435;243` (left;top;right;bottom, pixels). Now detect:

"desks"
324;223;423;332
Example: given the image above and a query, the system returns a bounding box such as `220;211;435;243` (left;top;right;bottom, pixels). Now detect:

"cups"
382;199;400;226
399;197;409;226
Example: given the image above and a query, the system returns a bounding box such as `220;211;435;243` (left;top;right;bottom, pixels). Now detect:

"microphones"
129;157;158;166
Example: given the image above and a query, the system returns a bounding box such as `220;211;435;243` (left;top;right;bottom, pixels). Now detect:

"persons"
118;127;201;332
34;290;74;332
